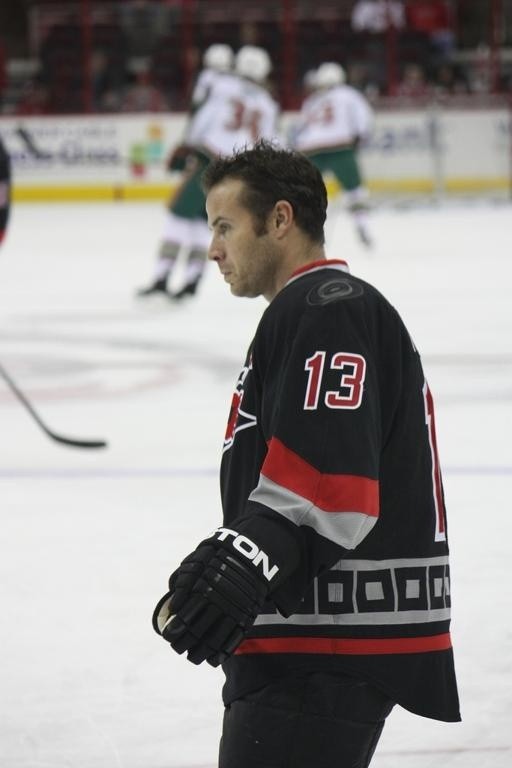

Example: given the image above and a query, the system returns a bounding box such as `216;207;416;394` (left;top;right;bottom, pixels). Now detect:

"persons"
188;42;234;114
1;137;16;259
290;58;381;252
154;138;462;766
304;73;327;108
136;45;286;303
1;1;511;116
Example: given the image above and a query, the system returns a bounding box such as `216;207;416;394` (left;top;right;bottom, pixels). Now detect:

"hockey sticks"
1;366;107;447
18;127;169;166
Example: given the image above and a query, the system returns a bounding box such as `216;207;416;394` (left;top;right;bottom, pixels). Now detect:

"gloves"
152;526;299;668
